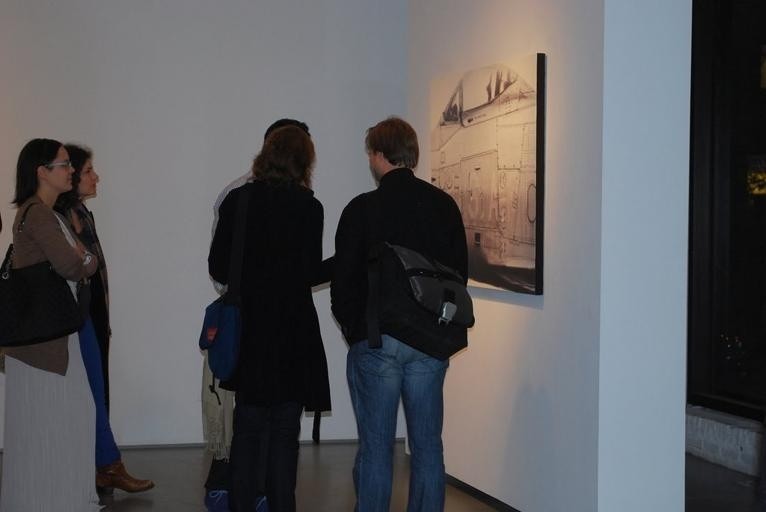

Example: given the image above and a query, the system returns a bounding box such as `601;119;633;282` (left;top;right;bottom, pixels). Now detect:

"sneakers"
205;490;228;512
256;496;267;512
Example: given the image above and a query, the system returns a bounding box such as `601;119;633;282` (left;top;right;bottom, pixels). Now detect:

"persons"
203;120;311;512
0;137;155;512
53;144;113;420
329;116;476;511
207;127;335;512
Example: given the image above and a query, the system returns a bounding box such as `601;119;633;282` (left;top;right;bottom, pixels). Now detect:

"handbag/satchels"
0;261;83;347
199;292;242;380
373;241;475;361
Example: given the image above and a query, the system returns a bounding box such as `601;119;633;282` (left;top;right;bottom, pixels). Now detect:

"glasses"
45;161;72;169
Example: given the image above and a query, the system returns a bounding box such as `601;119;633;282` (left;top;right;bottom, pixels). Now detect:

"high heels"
95;459;155;495
